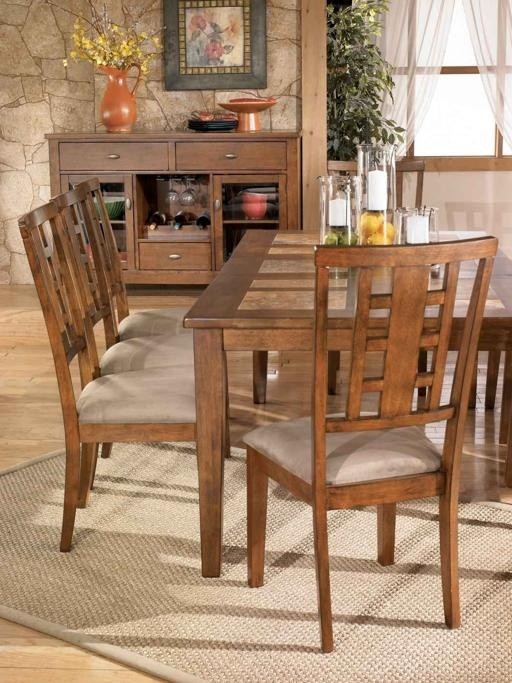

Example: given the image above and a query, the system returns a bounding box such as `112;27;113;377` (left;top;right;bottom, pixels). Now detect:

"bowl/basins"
241;202;268;221
241;193;268;203
93;196;125;218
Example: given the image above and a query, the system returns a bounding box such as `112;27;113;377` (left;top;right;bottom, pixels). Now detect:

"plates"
187;119;240;133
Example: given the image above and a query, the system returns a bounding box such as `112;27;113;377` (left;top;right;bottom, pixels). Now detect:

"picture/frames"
161;0;269;90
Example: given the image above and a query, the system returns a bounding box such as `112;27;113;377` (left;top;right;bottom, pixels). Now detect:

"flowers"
60;1;164;91
184;10;242;68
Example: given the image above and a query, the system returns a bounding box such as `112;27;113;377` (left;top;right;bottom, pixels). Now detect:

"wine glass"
163;179;197;206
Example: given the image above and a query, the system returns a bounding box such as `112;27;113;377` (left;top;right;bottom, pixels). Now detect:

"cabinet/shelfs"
46;131;302;291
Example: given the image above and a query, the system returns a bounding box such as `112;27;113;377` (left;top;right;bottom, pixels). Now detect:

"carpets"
0;403;512;683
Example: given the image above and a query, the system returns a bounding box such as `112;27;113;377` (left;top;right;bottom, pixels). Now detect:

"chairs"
327;160;424;242
50;190;233;459
72;176;217;341
241;232;498;651
18;200;223;555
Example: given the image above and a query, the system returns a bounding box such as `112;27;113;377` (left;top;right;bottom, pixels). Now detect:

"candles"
328;198;346;227
407;213;429;244
367;169;387;211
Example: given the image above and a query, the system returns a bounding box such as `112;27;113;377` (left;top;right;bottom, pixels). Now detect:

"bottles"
149;210;208;230
395;209;439;245
317;175;356;278
356;143;396;247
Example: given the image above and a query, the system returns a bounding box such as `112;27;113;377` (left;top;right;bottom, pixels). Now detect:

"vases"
96;62;145;135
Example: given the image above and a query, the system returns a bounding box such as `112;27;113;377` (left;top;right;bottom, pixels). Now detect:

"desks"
183;226;512;579
